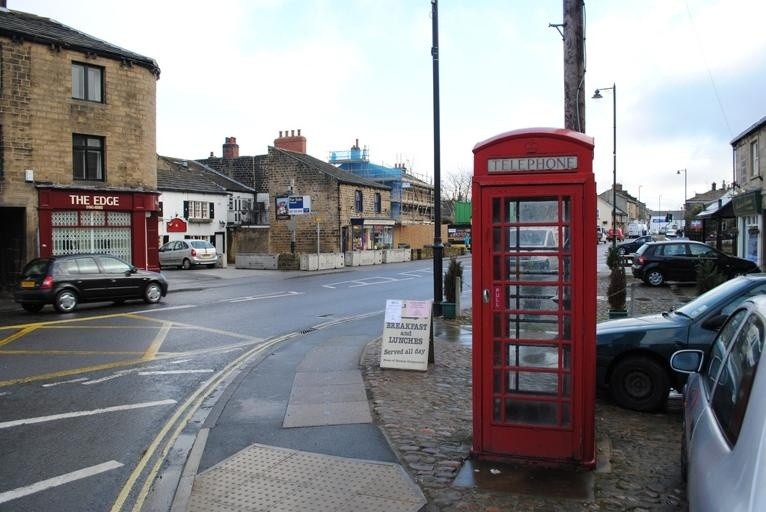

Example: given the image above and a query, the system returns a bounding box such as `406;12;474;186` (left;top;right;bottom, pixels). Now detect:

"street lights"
677;168;687;202
591;83;616;265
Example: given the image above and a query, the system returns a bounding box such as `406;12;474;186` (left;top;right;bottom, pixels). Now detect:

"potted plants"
607;261;628;318
439;255;465;319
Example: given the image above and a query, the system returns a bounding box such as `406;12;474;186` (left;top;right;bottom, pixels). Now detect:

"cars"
595;273;766;411
597;216;761;287
669;294;765;512
13;253;168;311
159;238;217;269
510;227;557;271
